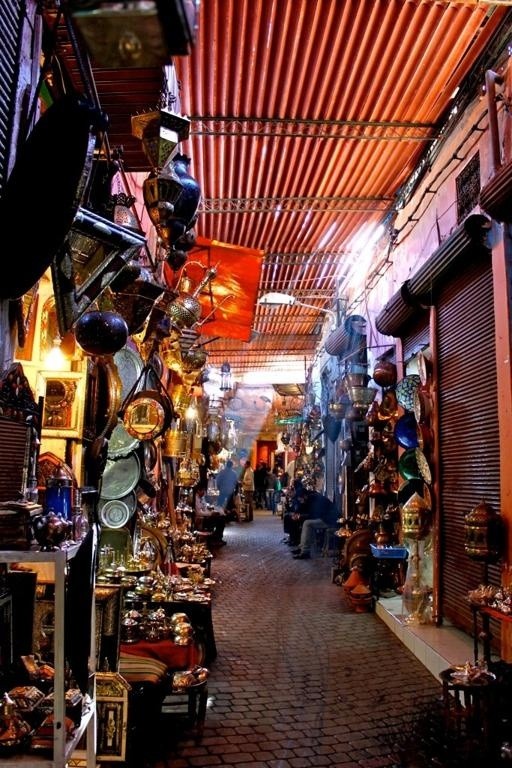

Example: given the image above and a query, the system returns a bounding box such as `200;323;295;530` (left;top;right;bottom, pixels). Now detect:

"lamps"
256;290;335;327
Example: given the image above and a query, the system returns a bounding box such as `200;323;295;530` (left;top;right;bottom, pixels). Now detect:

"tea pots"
166;259;236;329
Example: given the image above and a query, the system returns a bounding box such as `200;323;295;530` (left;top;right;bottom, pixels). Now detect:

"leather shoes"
293;552;311;559
291;548;301;554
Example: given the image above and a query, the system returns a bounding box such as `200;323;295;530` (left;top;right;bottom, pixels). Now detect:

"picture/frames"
34;370;88;440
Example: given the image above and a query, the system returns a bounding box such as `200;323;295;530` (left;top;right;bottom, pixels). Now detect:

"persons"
289;486;338;561
215;460;238;508
281;477;307;548
254;459;285;511
242;460;256;524
191;488;227;549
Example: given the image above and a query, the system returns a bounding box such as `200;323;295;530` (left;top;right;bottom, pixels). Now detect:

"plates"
98;346;166;530
396;351;433;507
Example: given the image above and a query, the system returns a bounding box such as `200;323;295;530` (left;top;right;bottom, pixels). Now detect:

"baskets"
370;544;407;559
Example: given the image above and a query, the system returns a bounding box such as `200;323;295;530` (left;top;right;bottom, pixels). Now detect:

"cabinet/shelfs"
0;521;99;768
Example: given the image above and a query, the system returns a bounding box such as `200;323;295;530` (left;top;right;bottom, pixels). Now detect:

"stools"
440;669;495;737
155;681;208;743
